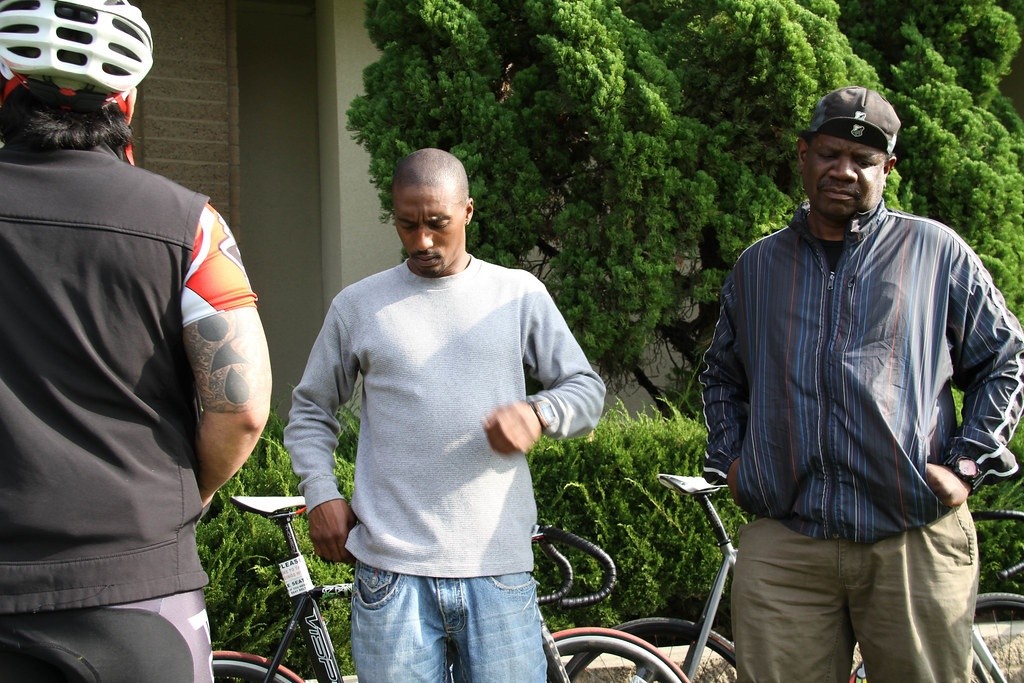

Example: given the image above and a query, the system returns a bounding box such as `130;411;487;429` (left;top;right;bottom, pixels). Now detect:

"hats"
809;85;901;155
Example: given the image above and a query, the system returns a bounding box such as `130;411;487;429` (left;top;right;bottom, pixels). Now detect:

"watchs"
527;399;558;436
942;454;983;493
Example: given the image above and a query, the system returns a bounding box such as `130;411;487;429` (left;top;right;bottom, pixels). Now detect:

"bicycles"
558;473;1023;683
208;496;695;682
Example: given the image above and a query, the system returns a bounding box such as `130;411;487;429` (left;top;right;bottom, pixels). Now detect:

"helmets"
0;0;155;113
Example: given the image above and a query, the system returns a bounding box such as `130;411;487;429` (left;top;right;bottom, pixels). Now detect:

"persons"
283;148;606;683
698;86;1023;683
0;0;273;683
